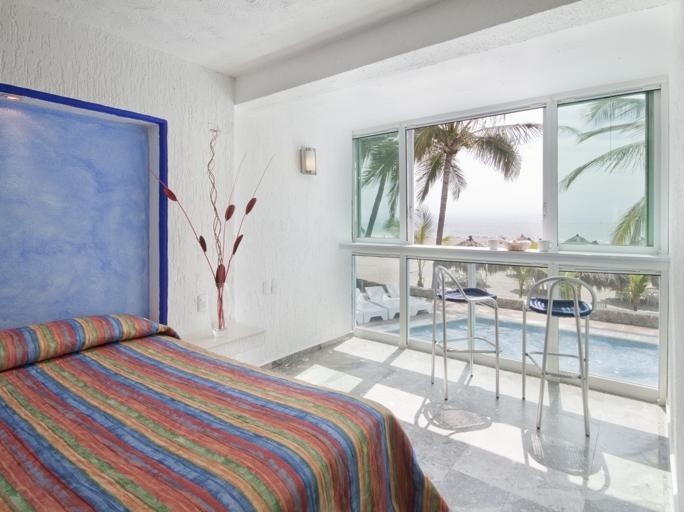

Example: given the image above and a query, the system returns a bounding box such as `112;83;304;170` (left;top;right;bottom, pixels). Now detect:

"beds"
0;314;457;512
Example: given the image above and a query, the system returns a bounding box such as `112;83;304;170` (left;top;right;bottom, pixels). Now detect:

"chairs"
356;288;388;324
522;274;597;438
431;264;500;401
385;281;431;317
364;286;400;320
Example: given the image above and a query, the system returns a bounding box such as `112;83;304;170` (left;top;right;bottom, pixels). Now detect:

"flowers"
146;120;278;330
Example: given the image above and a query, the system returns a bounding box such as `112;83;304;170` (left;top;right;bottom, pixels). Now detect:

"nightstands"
172;322;274;372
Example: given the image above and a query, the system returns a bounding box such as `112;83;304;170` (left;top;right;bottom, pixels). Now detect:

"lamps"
299;146;318;177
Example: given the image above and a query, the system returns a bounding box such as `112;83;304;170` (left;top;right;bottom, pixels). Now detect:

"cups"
537;240;554;253
488;238;498;251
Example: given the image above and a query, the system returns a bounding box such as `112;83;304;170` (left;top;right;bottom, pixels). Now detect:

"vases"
209;283;230;336
